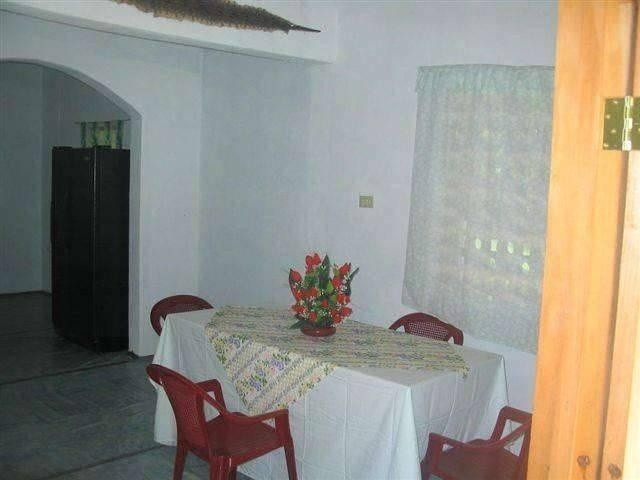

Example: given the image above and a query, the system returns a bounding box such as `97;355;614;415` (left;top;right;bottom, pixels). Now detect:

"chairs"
146;363;297;479
388;313;463;345
420;406;532;480
150;295;215;336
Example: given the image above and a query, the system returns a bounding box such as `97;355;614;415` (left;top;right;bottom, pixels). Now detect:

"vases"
301;327;335;336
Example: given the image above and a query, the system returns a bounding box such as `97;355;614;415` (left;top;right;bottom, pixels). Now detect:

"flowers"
287;252;360;330
